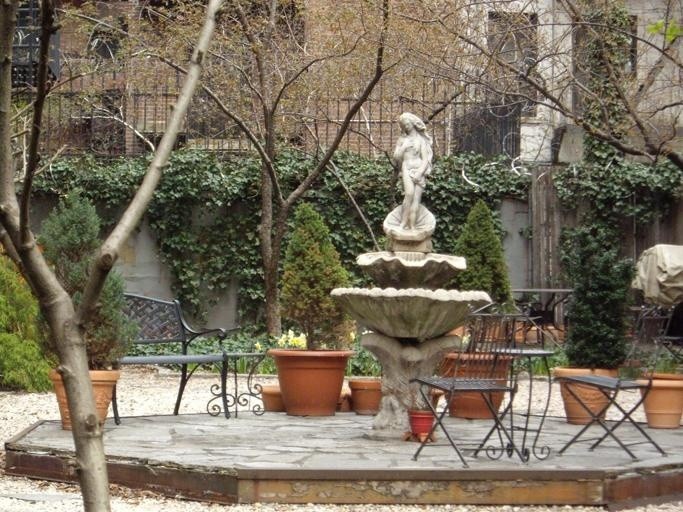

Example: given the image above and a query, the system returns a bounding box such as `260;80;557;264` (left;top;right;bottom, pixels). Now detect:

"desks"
471;349;561;460
510;287;574;346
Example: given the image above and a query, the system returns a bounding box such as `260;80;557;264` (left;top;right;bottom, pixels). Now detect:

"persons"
391;113;435;232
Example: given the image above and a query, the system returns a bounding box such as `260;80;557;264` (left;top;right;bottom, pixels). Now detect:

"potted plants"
444;199;516;418
406;386;436;434
552;225;637;425
643;358;682;380
267;198;355;416
35;186;137;430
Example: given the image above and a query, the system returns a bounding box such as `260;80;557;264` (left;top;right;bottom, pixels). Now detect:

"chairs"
509;302;543;347
408;304;531;467
114;291;267;424
553;305;676;464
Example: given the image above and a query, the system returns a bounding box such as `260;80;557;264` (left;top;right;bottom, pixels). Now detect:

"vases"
638;379;683;428
259;385;283;412
348;377;381;416
